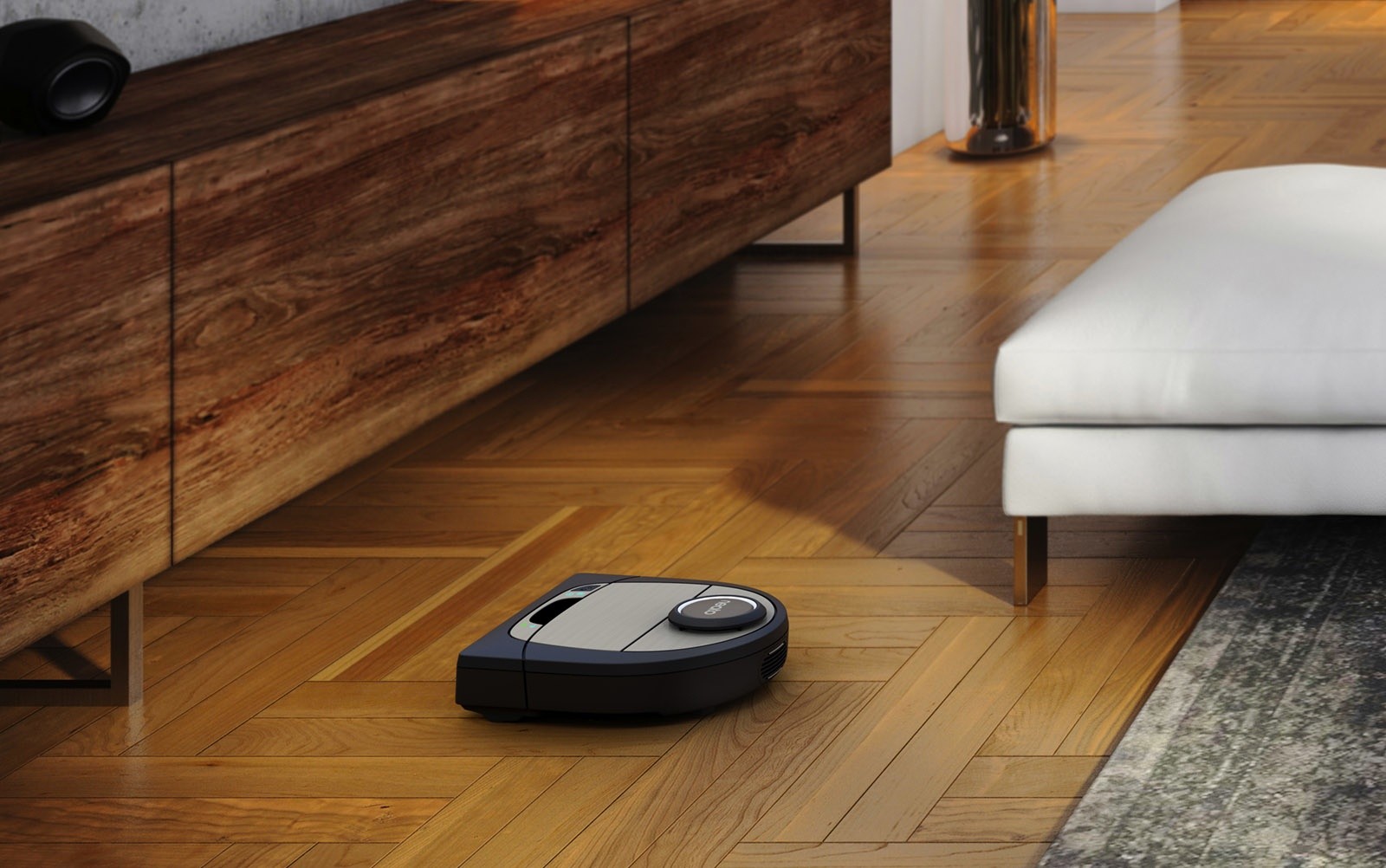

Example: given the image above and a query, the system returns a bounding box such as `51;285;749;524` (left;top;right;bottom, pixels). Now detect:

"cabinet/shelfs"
0;0;894;705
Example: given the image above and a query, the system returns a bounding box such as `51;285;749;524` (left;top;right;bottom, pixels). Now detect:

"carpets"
1037;515;1386;868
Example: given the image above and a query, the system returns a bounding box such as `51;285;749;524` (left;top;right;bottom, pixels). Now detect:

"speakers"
0;17;131;143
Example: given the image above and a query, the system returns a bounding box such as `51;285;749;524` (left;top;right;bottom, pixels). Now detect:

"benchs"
991;164;1386;606
